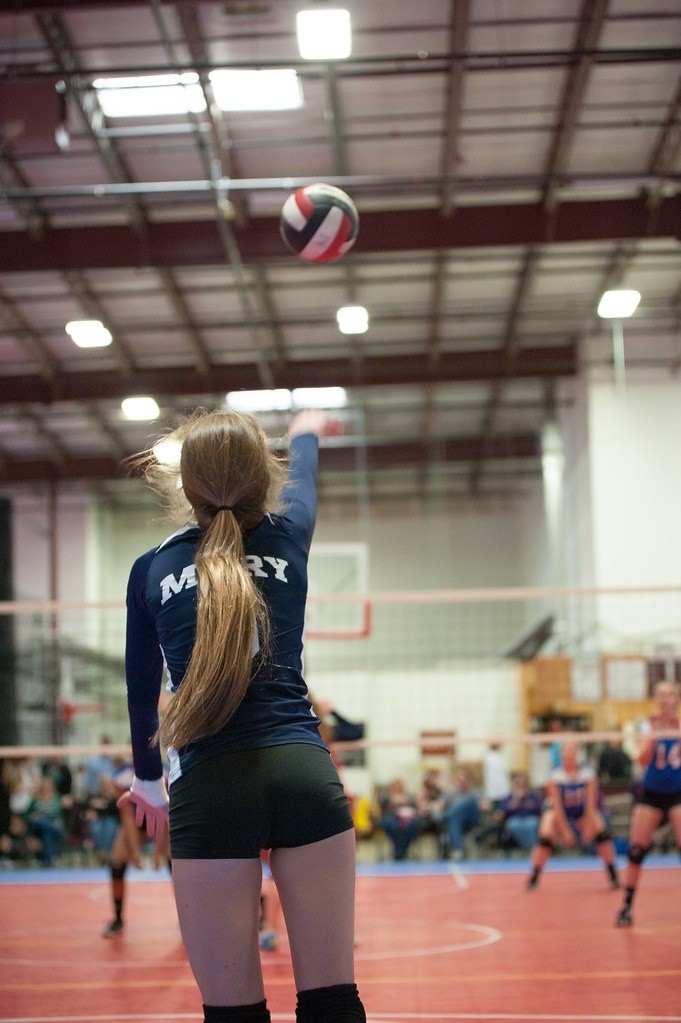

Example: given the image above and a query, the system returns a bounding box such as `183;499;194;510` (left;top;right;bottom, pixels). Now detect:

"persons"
616;681;681;928
311;695;673;863
527;735;619;892
116;410;368;1023
0;735;276;950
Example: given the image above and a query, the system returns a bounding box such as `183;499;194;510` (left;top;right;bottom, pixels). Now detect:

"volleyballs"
278;182;358;261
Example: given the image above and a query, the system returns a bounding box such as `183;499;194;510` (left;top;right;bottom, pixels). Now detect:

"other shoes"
617;910;630;925
104;921;126;939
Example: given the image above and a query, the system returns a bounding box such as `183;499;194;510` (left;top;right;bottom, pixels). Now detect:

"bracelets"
129;775;169;808
